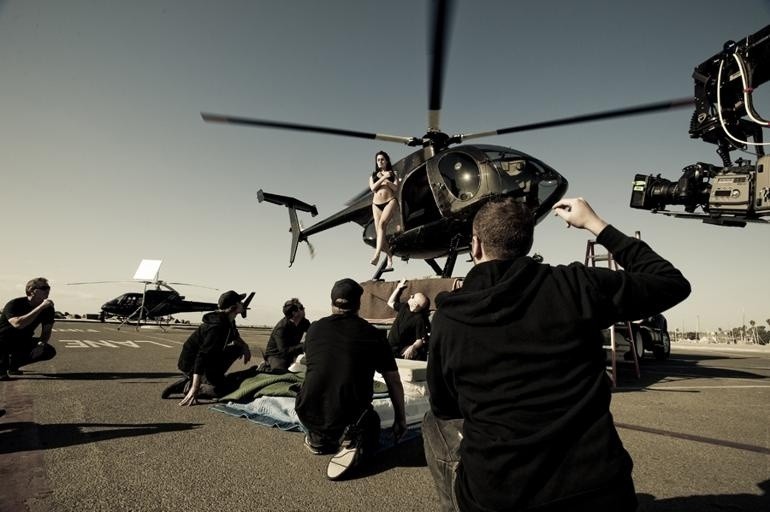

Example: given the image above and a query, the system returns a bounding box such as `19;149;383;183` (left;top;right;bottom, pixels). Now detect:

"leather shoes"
0;373;10;381
161;375;187;400
7;357;22;375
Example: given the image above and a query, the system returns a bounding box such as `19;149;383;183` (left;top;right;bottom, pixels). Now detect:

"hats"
217;291;246;311
330;278;364;310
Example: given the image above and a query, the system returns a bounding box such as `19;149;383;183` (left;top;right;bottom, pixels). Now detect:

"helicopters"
194;0;704;275
61;279;258;327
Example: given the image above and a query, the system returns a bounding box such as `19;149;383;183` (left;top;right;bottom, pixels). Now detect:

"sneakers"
326;427;362;481
302;431;326;455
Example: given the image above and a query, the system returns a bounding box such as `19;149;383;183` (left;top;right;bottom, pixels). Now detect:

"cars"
613;312;673;360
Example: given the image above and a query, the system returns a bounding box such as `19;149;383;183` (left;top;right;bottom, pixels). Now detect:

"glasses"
34;285;51;292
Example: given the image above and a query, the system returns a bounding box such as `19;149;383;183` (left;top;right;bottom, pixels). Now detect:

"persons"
385;278;430;359
421;196;693;512
263;298;311;371
369;151;400;269
178;291;251;407
0;278;56;380
294;277;407;480
